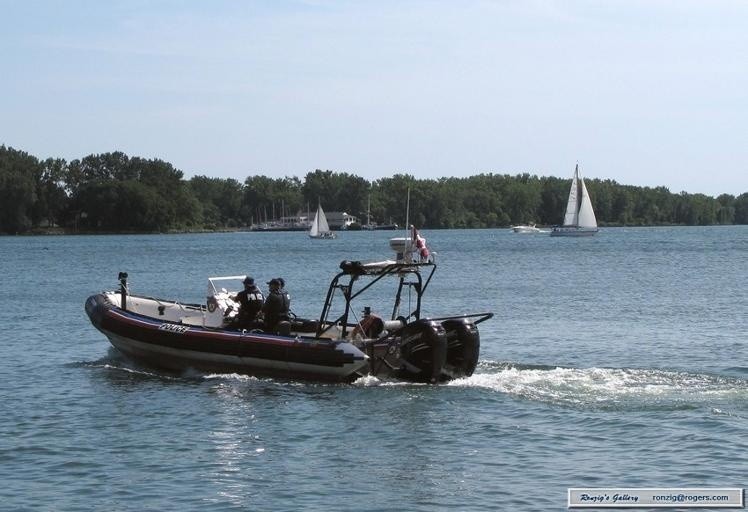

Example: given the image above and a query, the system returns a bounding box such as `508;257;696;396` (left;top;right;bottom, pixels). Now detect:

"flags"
410;225;430;259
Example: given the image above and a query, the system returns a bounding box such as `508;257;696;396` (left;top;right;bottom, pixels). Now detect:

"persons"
223;276;264;332
255;278;289;335
278;276;291;315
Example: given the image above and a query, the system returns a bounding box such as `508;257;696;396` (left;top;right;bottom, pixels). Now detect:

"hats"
265;277;286;286
241;277;256;287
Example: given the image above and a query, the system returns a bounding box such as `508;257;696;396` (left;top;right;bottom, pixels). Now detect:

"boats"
85;223;495;385
251;193;398;231
513;222;539;233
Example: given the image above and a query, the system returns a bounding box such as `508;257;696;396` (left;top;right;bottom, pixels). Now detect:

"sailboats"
309;196;337;240
550;163;599;236
